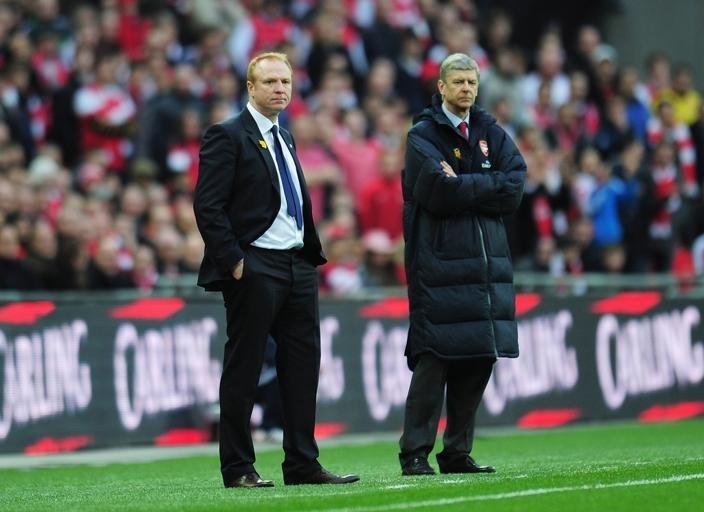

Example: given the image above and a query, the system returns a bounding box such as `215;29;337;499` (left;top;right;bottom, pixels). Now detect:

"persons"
192;52;364;490
1;0;704;292
398;51;527;476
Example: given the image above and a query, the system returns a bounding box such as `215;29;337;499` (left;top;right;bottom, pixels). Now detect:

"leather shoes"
439;455;497;474
286;466;361;485
228;471;275;488
402;457;437;475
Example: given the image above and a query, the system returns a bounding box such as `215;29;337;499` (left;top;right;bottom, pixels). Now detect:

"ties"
270;125;303;231
458;122;469;140
